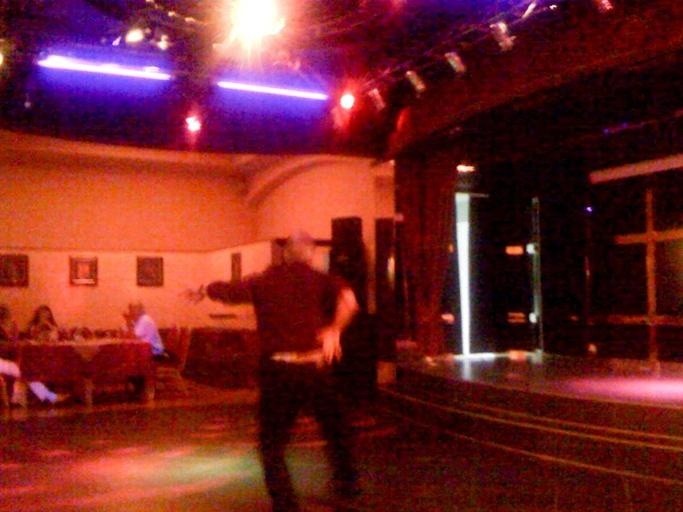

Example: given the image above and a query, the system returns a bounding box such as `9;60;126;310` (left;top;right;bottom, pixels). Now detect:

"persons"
28;304;65;340
0;302;67;406
119;301;165;399
177;230;361;512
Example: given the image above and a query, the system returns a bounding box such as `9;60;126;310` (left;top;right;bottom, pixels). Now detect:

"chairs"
0;325;193;408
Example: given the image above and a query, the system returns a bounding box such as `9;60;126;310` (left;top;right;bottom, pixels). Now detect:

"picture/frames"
136;255;164;287
69;254;99;288
0;254;29;288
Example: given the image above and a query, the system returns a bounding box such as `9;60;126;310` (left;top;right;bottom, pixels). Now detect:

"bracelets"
198;285;203;299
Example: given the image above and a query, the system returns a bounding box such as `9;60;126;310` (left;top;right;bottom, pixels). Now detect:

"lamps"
359;0;614;112
